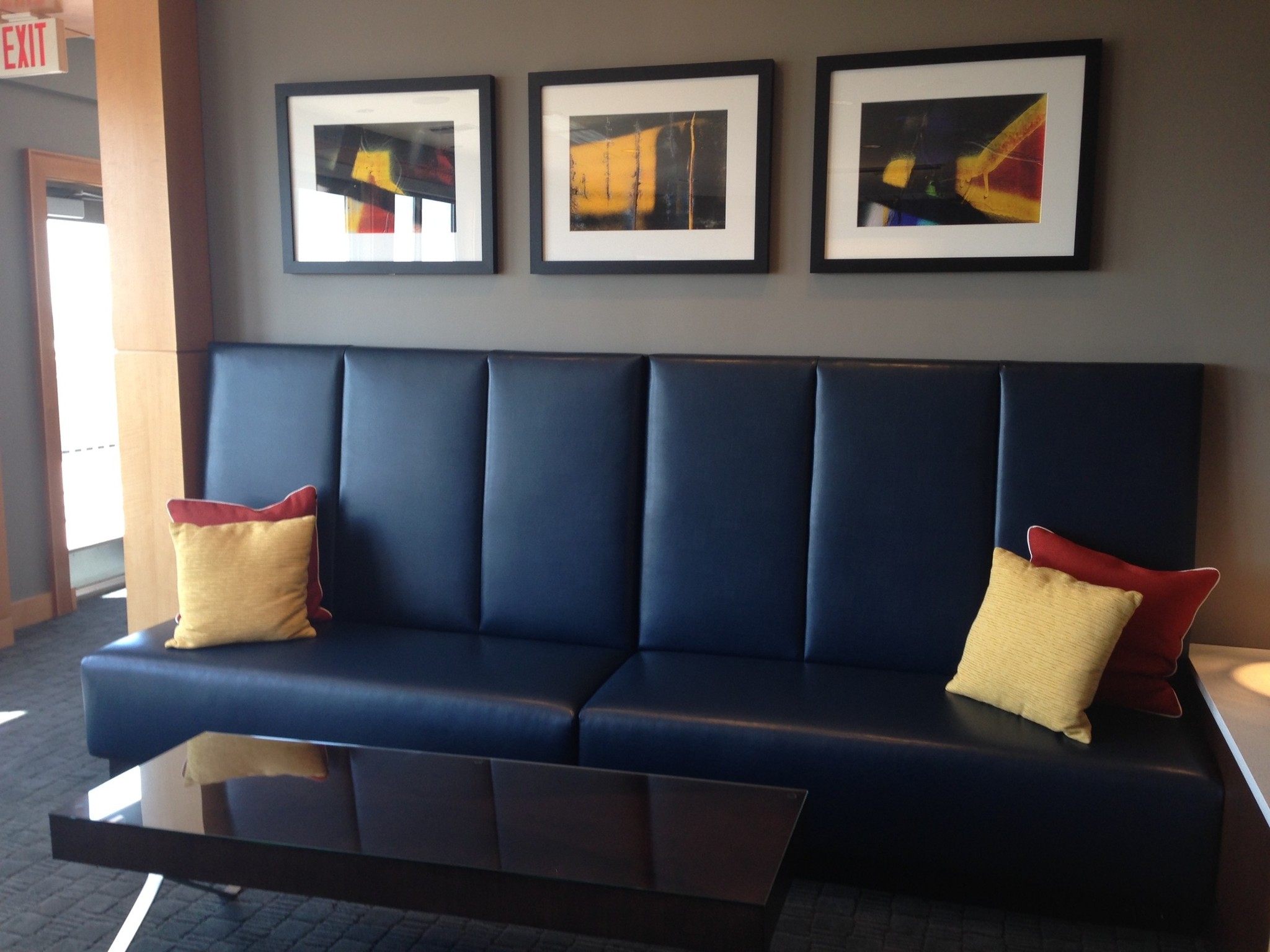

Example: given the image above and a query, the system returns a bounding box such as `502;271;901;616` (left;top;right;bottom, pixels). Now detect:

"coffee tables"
49;730;809;952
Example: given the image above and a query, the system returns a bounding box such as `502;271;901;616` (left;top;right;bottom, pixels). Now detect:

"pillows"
944;547;1145;744
1027;525;1221;718
167;485;332;625
169;516;317;649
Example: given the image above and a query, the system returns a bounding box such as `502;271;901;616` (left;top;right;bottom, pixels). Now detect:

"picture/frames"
811;40;1103;272
526;59;775;275
275;73;500;275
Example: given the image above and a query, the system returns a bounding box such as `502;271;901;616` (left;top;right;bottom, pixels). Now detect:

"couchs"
82;341;1223;925
